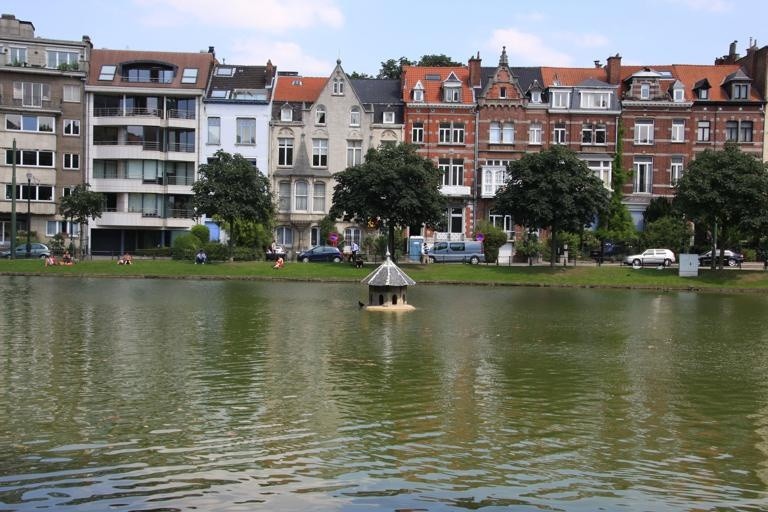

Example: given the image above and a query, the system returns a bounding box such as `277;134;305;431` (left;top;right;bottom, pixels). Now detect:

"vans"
420;241;486;264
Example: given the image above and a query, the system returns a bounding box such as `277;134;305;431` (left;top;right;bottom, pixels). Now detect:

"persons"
195;250;206;265
62;250;71;265
45;254;57;267
120;252;132;264
271;240;276;255
272;260;280;269
350;240;359;262
421;242;430;264
277;254;284;266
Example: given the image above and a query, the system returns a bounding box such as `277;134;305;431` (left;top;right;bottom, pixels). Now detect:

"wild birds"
358;300;364;308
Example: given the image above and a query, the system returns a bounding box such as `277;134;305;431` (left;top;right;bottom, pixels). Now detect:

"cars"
297;245;343;263
698;249;744;266
2;243;52;259
266;246;286;262
623;249;676;267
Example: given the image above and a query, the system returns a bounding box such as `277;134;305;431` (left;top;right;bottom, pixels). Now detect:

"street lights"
25;173;33;259
